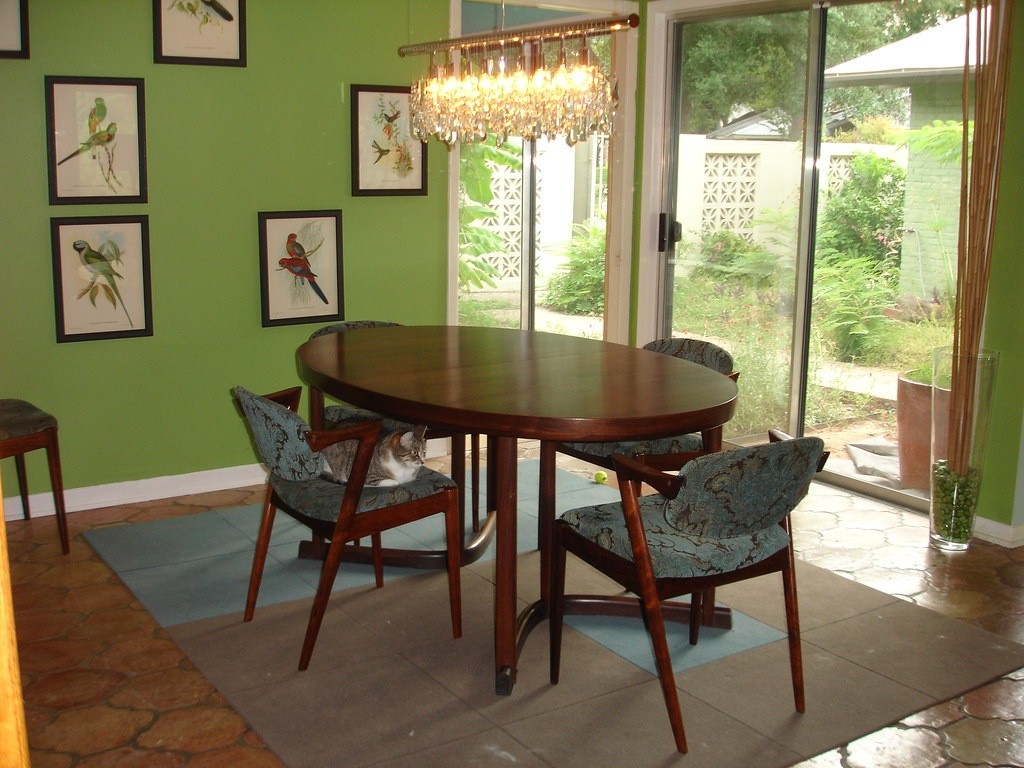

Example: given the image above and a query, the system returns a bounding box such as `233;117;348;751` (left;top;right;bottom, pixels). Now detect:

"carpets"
81;457;1023;768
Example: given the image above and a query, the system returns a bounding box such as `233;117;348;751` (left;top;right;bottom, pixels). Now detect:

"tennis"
595;470;608;484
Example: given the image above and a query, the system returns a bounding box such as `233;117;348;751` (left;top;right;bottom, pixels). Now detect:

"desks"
296;325;739;697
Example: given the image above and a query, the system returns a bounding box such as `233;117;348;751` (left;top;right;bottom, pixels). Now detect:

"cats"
319;423;429;488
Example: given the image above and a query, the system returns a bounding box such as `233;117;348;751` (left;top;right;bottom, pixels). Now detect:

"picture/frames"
349;84;428;198
49;214;155;344
44;74;148;205
0;0;31;60
257;208;345;328
152;0;247;68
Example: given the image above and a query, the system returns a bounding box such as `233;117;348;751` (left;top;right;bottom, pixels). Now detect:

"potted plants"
896;293;956;489
930;0;1016;551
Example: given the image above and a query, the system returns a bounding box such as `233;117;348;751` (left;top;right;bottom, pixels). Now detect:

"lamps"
399;0;640;153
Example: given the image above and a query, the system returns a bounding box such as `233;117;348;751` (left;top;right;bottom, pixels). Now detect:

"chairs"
0;399;70;555
308;320;480;589
555;336;741;496
548;429;831;755
230;384;462;670
